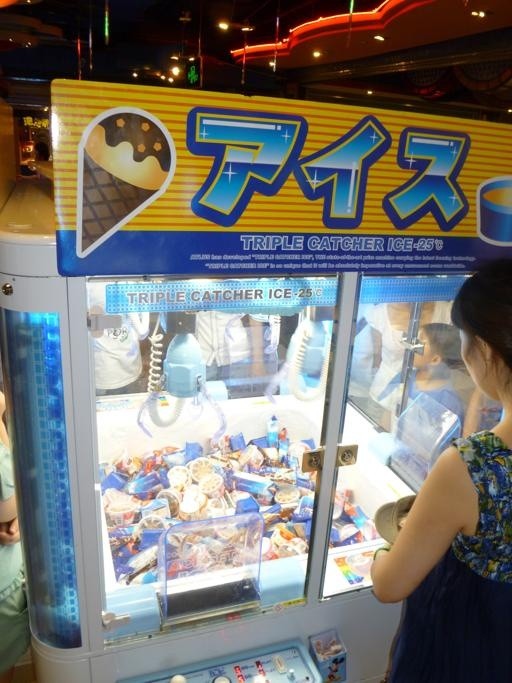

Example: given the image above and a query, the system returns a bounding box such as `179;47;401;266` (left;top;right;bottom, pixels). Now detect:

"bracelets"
373;547;390;561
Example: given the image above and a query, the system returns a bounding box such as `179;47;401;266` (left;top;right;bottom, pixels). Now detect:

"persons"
82;278;154;398
367;272;464;440
183;275;283;399
372;320;482;473
1;391;40;682
367;254;512;683
